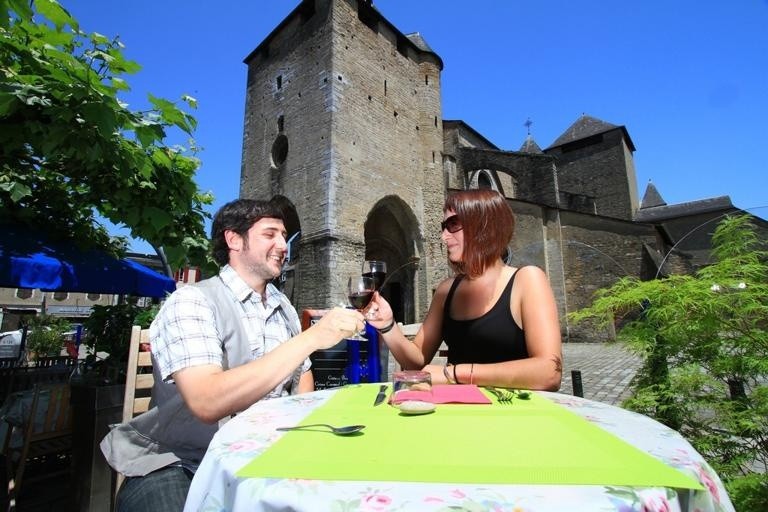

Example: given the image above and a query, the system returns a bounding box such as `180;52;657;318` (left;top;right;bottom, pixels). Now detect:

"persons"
362;189;562;391
98;199;367;511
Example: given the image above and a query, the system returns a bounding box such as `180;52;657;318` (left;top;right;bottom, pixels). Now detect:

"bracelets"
453;362;461;384
375;318;395;335
444;362;456;384
470;363;473;385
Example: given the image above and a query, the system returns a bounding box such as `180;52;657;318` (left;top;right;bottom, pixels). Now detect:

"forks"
485;385;515;401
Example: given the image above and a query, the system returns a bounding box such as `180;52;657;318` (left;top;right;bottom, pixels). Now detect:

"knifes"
373;385;388;406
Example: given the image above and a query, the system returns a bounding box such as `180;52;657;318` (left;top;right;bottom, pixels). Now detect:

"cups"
392;370;432;409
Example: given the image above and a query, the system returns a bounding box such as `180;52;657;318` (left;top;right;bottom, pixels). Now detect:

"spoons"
507;389;529;399
276;424;366;435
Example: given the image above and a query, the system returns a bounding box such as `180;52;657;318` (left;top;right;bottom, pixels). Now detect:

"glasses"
441;213;463;235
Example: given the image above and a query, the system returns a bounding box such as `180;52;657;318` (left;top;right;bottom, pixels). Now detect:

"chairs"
113;317;452;497
0;355;82;501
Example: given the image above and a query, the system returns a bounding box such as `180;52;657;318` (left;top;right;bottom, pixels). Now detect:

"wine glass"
342;275;375;343
362;260;386;322
